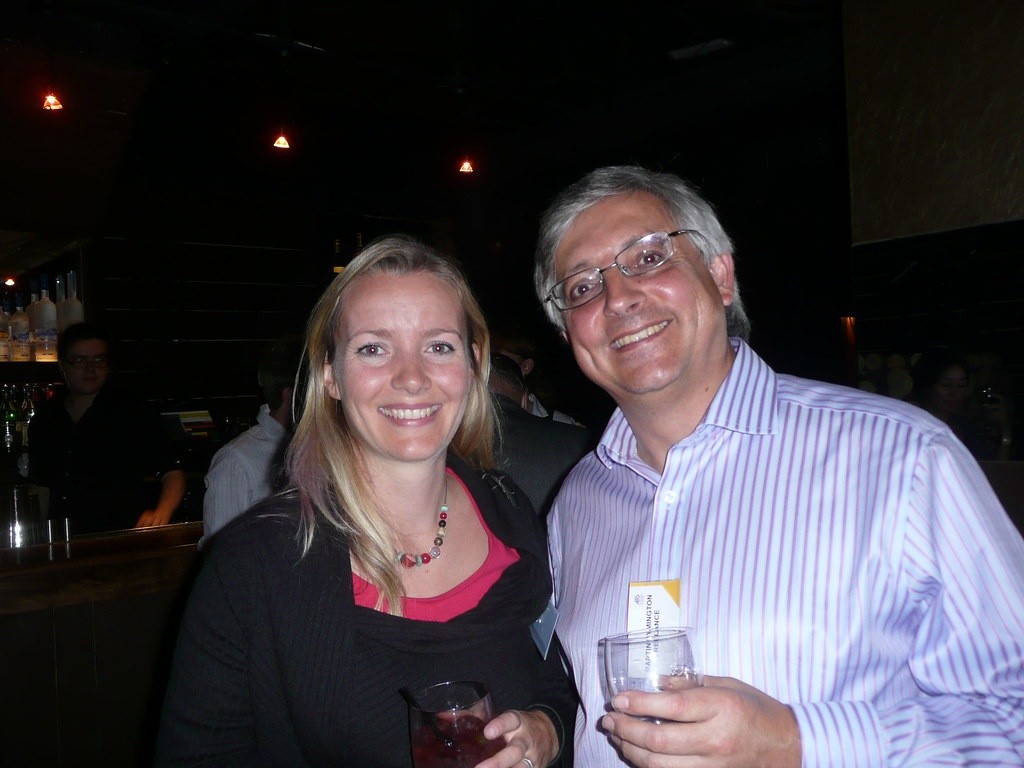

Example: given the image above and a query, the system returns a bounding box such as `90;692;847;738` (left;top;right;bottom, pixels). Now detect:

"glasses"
61;353;108;370
544;230;707;311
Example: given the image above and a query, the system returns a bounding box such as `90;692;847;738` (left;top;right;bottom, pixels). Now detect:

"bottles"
0;382;56;458
0;271;84;363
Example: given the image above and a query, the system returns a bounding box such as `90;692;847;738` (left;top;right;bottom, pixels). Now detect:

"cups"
409;680;507;768
605;626;706;725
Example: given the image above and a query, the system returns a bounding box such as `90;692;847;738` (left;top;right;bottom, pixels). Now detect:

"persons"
151;238;601;768
534;166;1024;768
27;323;185;536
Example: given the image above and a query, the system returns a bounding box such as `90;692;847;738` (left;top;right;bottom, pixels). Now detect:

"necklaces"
392;477;447;569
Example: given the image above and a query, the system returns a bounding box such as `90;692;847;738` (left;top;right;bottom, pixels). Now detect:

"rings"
521;757;534;768
